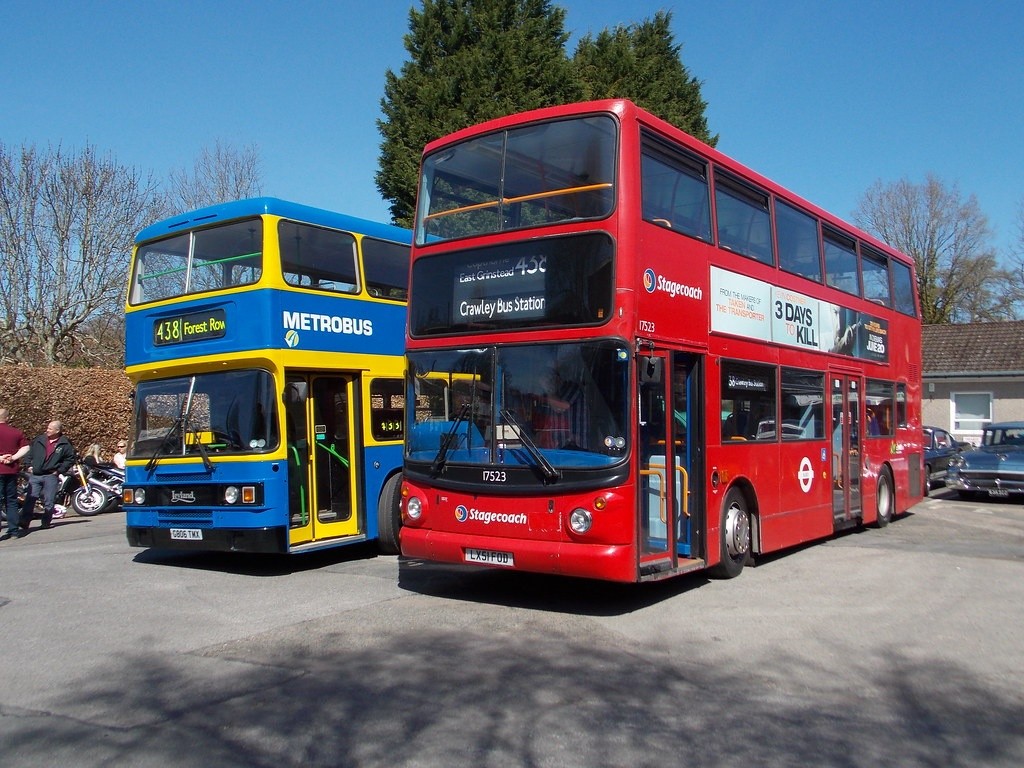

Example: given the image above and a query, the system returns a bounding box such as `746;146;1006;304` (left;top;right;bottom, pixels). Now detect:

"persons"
526;356;591;451
0;408;31;540
19;419;76;541
83;440;128;470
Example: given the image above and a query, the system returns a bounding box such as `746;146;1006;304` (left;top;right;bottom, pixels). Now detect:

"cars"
922;425;971;498
944;421;1024;502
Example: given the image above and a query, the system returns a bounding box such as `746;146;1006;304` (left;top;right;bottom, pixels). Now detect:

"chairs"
781;423;807;439
755;420;776;440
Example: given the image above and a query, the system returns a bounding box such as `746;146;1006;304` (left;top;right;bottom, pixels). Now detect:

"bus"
122;196;491;558
398;99;925;586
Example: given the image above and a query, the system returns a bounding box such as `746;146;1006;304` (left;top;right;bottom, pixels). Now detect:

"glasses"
118;446;125;447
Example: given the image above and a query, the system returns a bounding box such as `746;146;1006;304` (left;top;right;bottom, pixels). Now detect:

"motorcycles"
0;449;125;522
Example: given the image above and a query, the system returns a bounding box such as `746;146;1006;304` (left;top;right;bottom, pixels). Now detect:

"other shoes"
9;529;19;539
41;523;51;528
18;520;30;528
